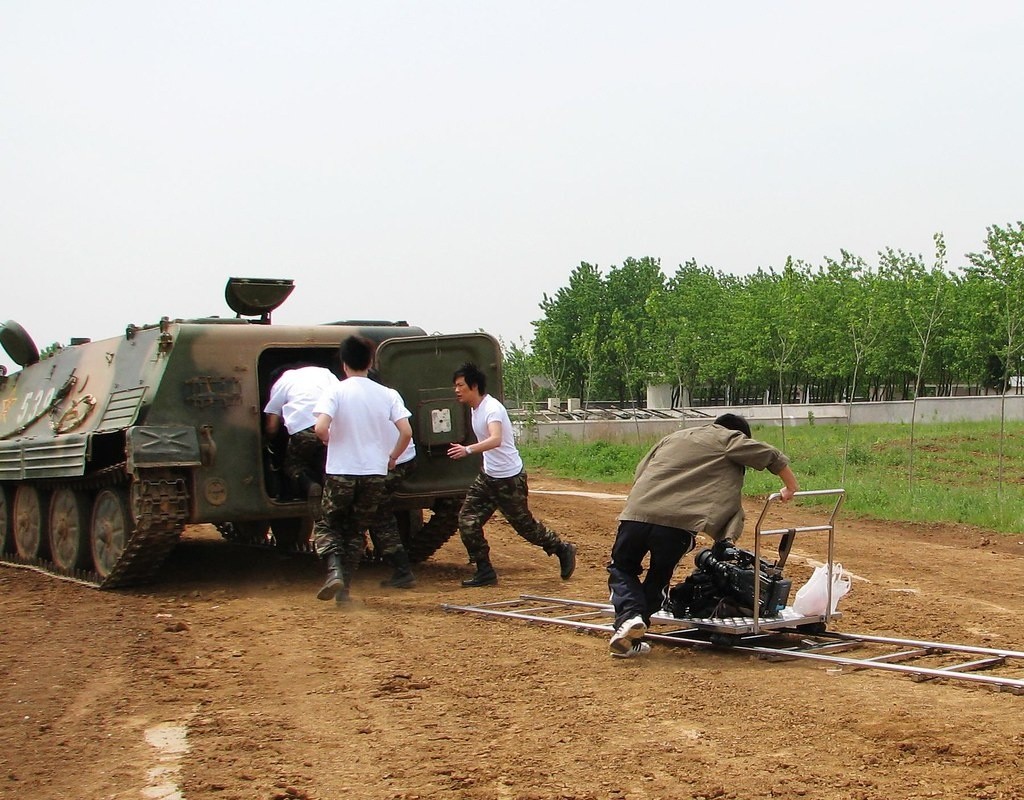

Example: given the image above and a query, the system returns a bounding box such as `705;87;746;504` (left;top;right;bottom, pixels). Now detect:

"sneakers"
609;614;647;654
610;641;652;658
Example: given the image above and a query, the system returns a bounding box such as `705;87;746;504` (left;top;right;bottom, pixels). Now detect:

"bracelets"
465;446;472;455
390;456;397;460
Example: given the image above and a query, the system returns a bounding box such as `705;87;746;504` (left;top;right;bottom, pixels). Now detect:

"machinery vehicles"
0;279;507;590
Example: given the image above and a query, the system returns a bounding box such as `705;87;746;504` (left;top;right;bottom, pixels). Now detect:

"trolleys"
605;488;846;642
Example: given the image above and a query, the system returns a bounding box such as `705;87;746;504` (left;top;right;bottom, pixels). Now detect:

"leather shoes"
556;542;577;580
461;569;498;588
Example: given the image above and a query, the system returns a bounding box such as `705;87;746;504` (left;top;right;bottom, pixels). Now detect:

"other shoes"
316;570;345;601
306;482;322;521
380;572;417;588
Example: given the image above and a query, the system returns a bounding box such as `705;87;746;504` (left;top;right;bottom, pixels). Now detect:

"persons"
447;363;576;587
369;421;419;587
264;365;341;504
314;336;413;600
607;415;797;658
